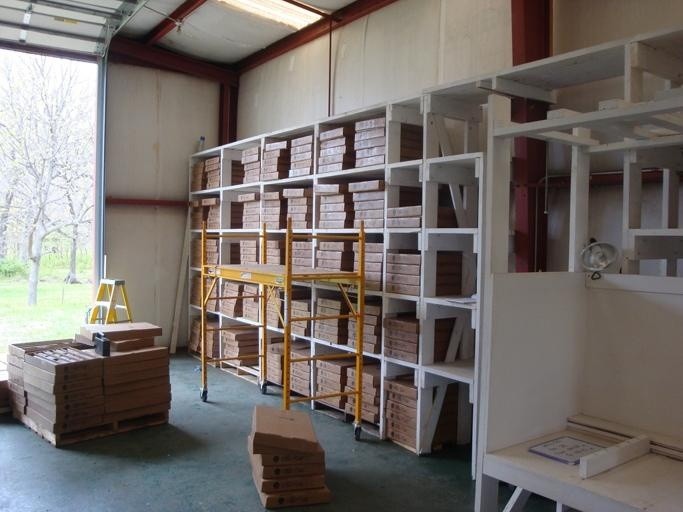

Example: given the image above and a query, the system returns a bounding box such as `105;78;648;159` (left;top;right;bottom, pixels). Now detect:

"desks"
474;426;683;512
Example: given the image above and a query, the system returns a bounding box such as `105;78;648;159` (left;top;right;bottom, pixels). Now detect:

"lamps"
580;237;618;281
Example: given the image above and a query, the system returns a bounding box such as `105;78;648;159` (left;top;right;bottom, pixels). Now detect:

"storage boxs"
6;321;173;436
241;404;332;511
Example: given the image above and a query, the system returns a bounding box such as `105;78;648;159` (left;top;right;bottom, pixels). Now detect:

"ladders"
88;279;133;325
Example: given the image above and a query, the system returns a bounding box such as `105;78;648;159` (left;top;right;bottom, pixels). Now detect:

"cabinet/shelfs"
496;24;683;164
188;77;492;455
